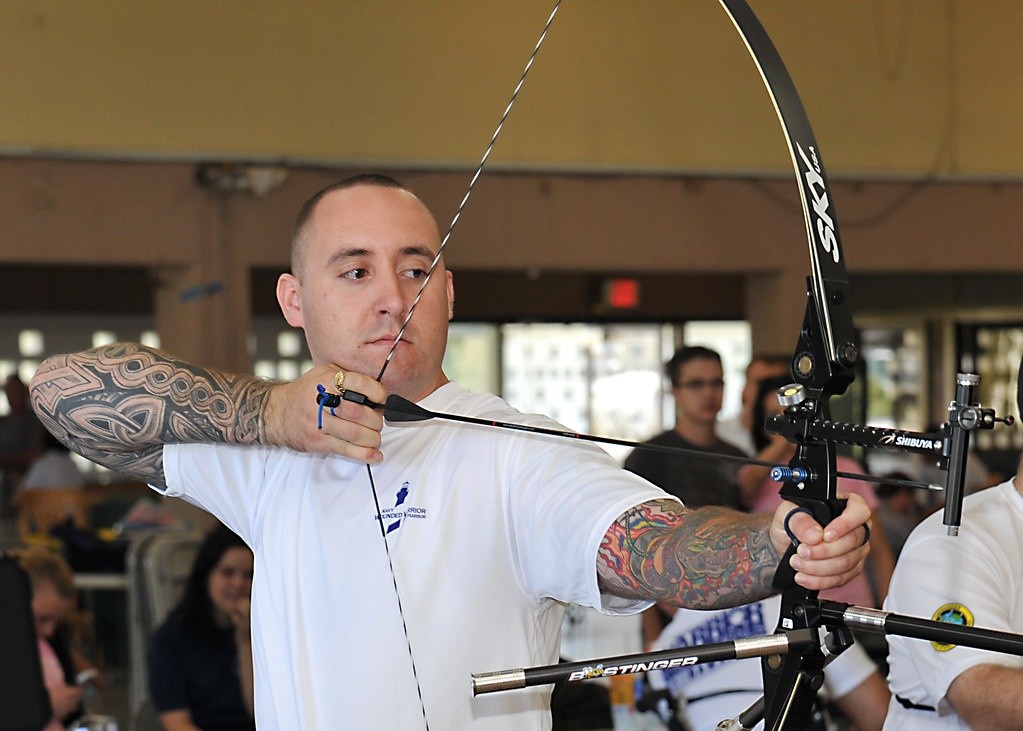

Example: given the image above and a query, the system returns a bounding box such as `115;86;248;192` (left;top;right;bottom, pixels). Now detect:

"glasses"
677;379;724;389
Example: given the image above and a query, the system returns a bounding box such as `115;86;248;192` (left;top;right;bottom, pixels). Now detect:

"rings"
333;372;348;391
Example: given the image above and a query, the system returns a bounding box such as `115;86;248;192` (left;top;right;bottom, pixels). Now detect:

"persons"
0;344;1023;731
29;171;877;731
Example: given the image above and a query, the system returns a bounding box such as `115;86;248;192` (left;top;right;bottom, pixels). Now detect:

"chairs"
122;532;200;730
